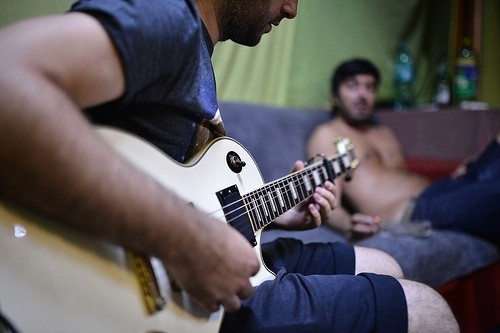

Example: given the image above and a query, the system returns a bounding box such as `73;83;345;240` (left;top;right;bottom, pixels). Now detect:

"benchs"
214;99;500;333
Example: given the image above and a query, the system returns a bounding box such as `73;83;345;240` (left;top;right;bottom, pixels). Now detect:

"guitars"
0;125;359;332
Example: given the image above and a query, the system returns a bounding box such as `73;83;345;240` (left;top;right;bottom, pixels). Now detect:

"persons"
307;57;500;239
0;0;460;333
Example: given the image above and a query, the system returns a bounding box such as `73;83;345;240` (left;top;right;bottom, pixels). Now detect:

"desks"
374;108;500;161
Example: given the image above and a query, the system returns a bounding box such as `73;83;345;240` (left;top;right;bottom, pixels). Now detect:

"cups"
432;77;451;107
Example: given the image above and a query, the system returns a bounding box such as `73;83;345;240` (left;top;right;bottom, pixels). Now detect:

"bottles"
455;34;478;105
392;44;416;111
